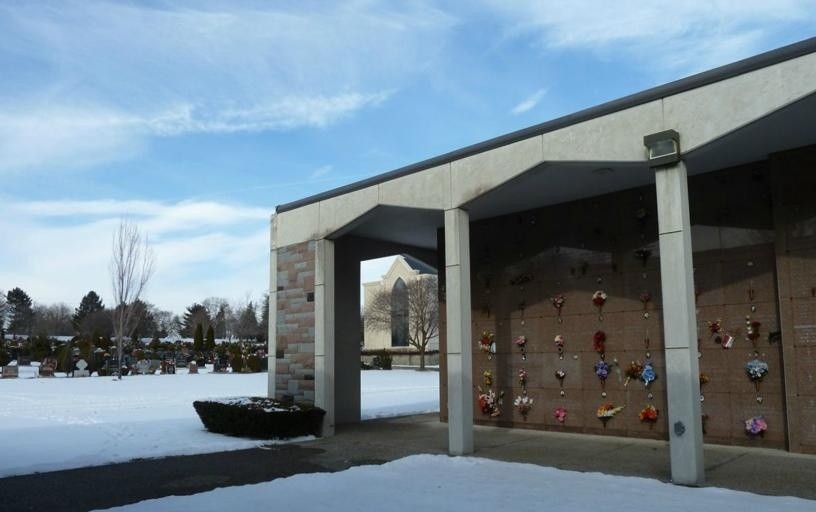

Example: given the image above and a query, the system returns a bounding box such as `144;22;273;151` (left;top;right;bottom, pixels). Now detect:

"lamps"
643;129;681;168
